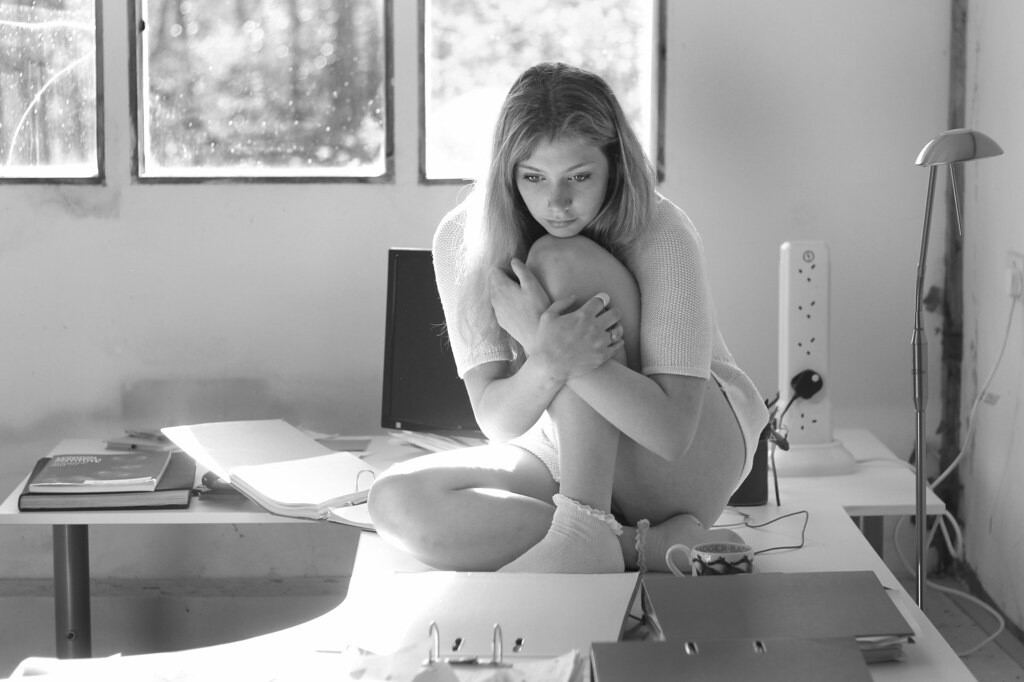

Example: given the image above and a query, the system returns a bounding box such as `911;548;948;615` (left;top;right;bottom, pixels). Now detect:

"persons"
366;63;771;571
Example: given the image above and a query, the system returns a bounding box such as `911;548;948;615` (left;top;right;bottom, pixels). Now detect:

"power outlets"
1005;251;1024;304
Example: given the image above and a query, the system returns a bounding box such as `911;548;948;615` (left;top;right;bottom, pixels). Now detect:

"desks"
0;425;945;658
8;506;976;682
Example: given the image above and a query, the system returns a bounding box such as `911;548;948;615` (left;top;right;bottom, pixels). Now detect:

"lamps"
909;129;1004;609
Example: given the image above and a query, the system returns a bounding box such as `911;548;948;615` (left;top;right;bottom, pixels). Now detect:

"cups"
665;542;754;576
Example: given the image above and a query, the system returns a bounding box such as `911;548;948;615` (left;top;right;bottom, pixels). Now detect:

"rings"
608;327;617;341
596;289;608;305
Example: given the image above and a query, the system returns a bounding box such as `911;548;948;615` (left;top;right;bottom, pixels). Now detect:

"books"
17;452;197;512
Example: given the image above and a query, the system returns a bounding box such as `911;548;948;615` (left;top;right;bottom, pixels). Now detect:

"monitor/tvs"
381;249;490;440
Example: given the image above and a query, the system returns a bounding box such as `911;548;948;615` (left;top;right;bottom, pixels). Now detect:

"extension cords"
778;243;833;444
